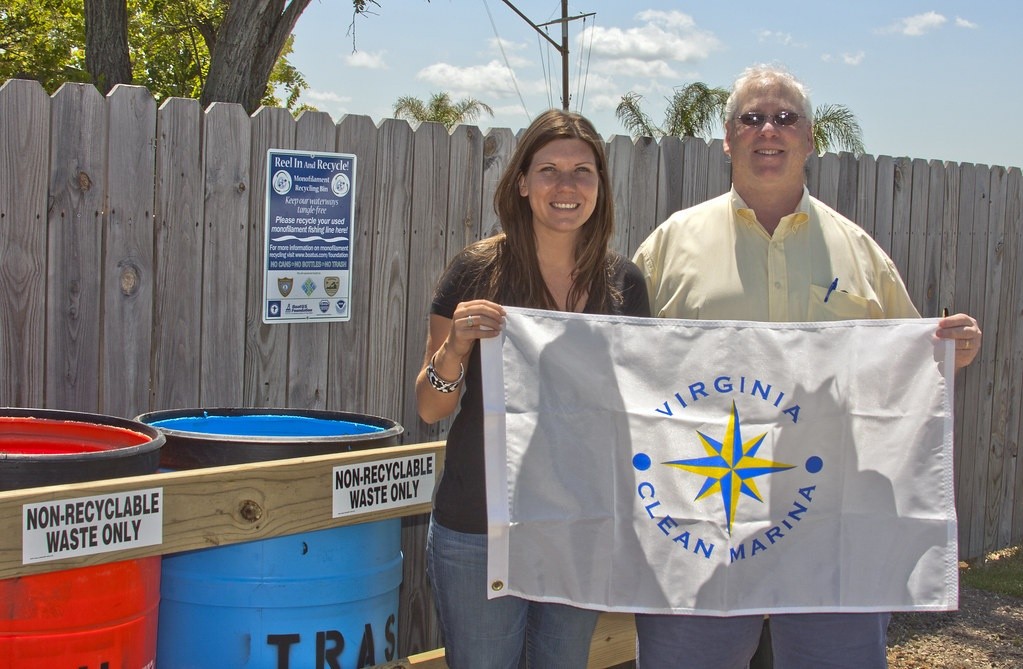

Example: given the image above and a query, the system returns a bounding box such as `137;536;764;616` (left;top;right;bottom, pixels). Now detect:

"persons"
416;110;655;669
629;65;984;669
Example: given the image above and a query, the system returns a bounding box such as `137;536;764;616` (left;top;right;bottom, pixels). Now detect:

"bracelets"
426;351;464;393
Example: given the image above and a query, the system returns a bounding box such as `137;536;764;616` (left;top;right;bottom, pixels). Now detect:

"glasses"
731;110;807;127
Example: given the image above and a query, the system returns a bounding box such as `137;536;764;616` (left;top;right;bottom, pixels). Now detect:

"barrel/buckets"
0;407;168;669
132;407;406;669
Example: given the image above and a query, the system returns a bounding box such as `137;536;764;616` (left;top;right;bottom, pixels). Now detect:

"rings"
468;316;474;326
965;340;969;349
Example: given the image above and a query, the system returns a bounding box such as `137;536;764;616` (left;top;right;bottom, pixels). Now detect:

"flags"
480;304;959;617
